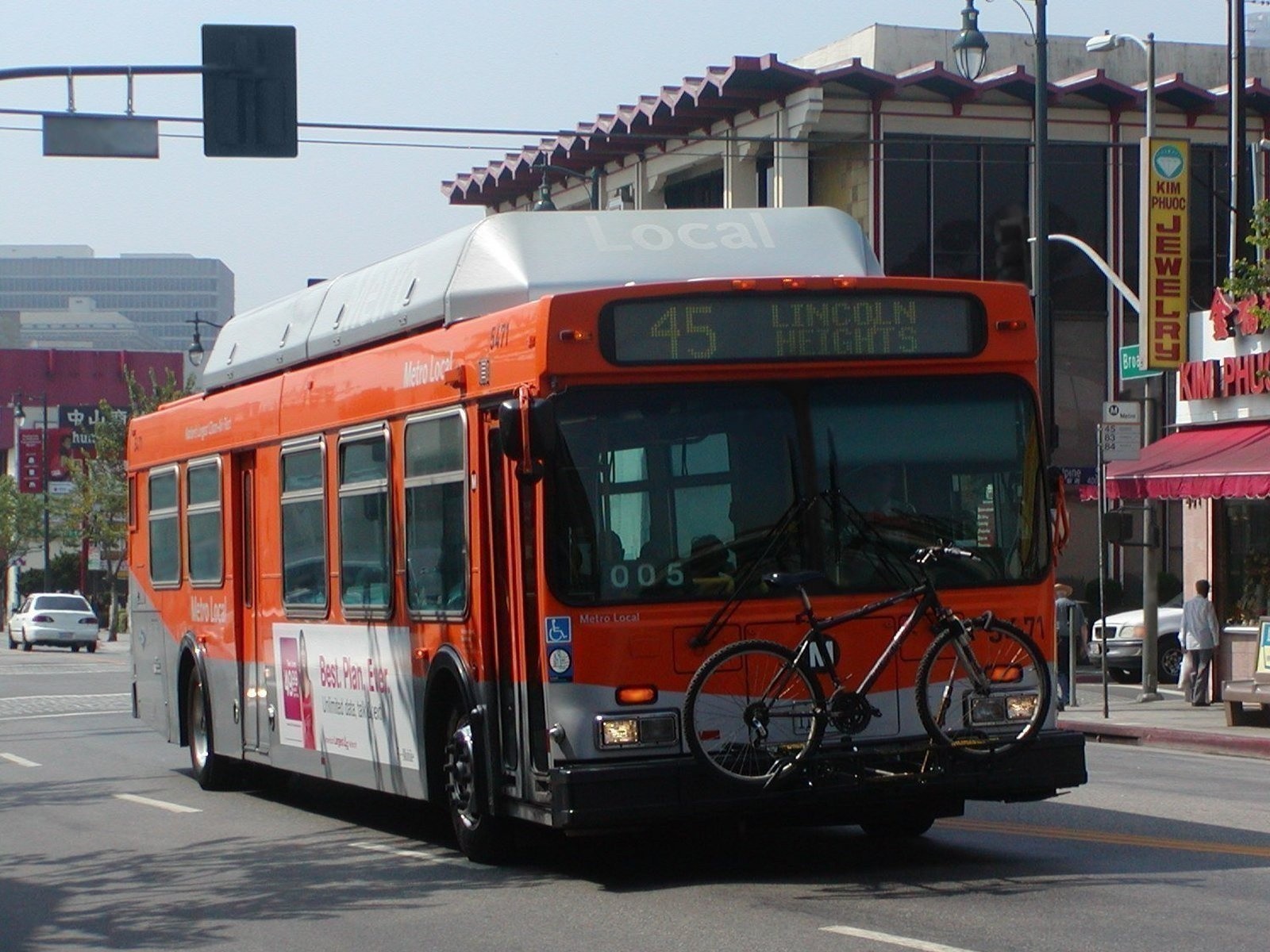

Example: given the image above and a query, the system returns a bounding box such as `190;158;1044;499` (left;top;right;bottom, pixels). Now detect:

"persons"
1178;579;1220;707
1054;582;1089;705
603;530;735;581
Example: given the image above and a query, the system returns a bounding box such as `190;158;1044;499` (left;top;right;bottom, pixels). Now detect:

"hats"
1054;583;1073;597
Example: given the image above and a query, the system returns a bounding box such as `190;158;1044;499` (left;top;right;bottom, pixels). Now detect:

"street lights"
1085;30;1154;701
953;1;1053;479
12;386;47;590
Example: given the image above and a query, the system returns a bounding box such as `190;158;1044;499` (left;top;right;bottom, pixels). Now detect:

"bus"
127;206;1089;868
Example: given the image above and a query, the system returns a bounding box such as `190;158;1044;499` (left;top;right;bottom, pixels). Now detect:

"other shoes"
1192;702;1210;706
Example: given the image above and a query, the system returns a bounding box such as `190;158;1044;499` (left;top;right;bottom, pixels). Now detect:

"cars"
1085;565;1264;685
8;593;99;653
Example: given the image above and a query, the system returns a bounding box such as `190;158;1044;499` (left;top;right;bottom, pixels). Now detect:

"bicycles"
681;537;1052;791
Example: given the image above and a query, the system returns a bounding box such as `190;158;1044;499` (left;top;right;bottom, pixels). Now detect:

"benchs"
1220;616;1270;726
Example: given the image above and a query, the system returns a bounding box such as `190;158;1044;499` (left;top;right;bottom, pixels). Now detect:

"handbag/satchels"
1075;635;1091;666
1177;650;1194;690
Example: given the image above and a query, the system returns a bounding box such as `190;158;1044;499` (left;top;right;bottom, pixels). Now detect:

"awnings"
116;572;128;579
1079;424;1270;501
